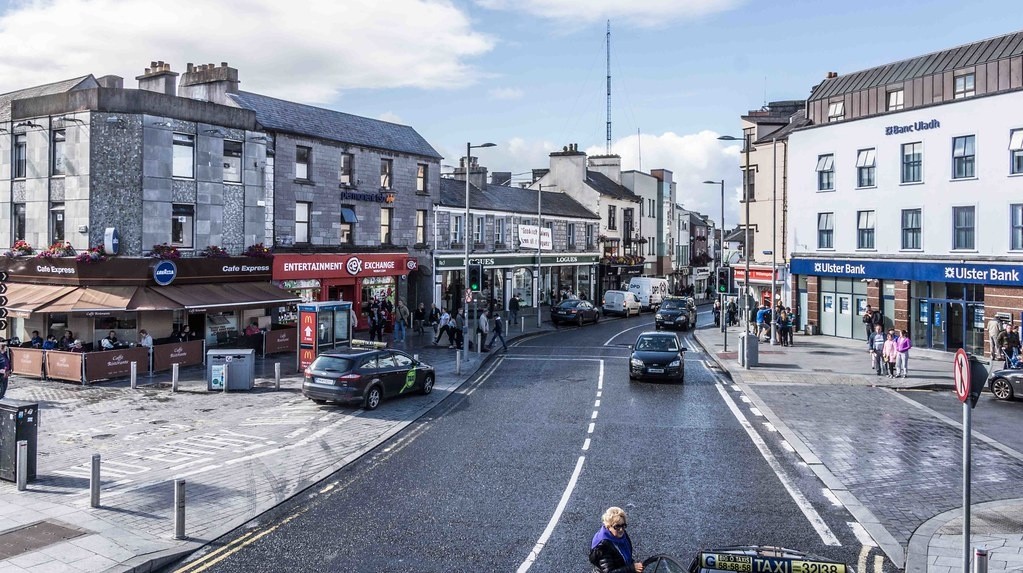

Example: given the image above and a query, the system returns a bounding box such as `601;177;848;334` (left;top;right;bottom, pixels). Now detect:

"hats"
493;313;499;316
757;305;765;308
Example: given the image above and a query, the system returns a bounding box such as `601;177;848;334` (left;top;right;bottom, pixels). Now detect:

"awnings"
0;283;302;319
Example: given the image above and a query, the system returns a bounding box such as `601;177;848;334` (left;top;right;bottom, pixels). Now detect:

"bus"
551;298;600;327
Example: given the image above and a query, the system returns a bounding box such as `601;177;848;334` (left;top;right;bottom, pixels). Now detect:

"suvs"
655;296;698;331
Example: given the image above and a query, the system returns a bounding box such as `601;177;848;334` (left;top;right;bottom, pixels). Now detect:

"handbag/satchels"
405;320;408;325
883;356;890;363
476;328;483;335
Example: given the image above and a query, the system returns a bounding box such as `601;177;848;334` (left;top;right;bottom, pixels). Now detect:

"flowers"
3;240;32;257
144;242;183;261
35;241;77;261
244;243;275;258
200;244;232;259
75;243;108;265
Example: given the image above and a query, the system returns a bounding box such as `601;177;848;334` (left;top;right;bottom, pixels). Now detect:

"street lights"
717;135;750;371
536;184;557;328
702;180;724;332
463;143;498;361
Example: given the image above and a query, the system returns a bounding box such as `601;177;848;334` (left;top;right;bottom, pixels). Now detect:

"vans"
628;277;670;312
602;291;643;318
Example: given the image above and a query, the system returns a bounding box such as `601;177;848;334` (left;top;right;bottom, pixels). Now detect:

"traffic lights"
717;267;730;294
468;264;482;292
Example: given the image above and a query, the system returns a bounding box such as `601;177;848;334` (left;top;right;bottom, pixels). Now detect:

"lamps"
249;136;273;142
11;123;42;128
50;117;81;122
106;116;127;124
204;129;227;135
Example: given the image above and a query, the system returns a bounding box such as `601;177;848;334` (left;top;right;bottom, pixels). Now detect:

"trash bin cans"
207;348;255;390
738;333;759;365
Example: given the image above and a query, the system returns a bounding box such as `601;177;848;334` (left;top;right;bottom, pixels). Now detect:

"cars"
987;361;1023;401
301;344;436;411
641;544;848;573
627;331;688;381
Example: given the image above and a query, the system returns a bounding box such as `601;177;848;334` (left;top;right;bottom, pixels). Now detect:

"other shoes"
393;340;398;342
758;340;763;344
895;375;907;378
400;340;404;342
886;375;894;378
449;345;454;348
763;334;770;338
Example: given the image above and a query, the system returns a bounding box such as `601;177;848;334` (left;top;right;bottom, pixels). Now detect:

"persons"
668;281;797;348
0;330;261;400
350;285;588;353
861;305;911;379
987;315;1023;370
589;507;643;573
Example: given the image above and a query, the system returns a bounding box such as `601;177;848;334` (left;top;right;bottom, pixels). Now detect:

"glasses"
611;523;627;530
